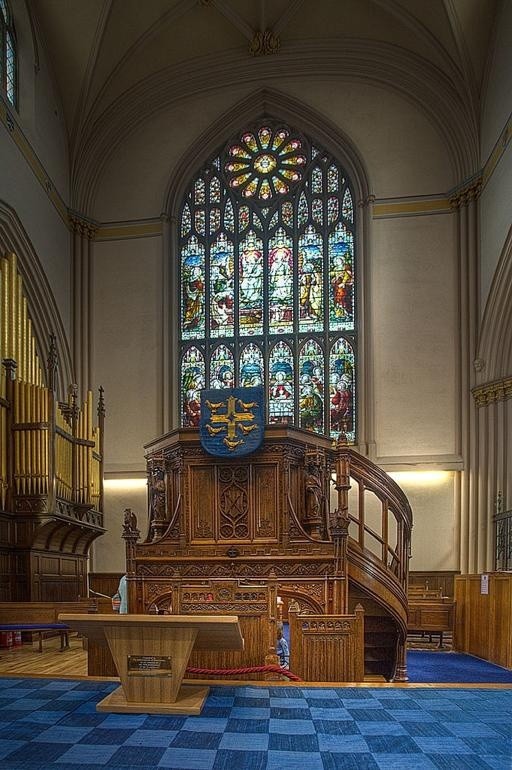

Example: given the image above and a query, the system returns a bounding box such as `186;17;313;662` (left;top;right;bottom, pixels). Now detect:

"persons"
183;241;352;434
116;571;128;614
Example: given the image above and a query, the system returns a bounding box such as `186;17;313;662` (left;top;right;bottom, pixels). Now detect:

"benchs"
407;581;456;650
0;625;71;653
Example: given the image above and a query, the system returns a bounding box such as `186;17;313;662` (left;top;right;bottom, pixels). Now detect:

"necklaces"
276;620;289;681
